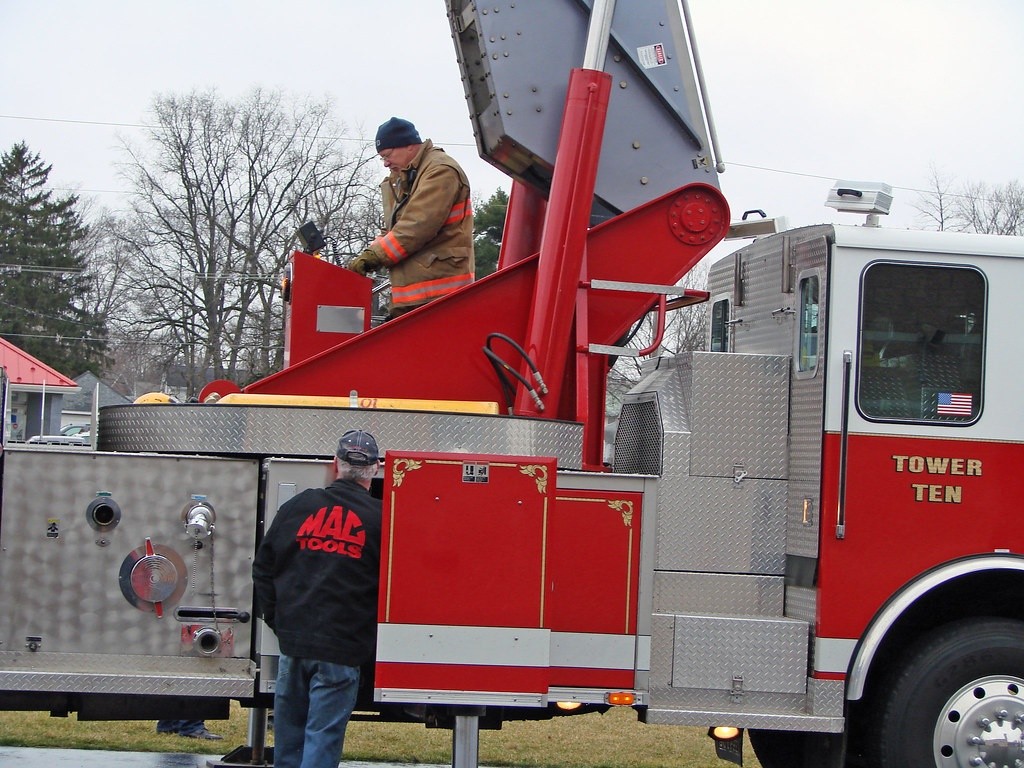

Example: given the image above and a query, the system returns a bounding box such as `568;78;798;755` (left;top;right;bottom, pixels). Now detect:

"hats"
335;430;378;466
375;117;422;152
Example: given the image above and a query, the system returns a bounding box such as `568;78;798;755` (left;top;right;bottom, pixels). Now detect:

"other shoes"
267;715;274;732
185;728;223;739
157;727;180;734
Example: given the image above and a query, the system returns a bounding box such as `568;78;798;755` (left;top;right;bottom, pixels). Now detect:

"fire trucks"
0;0;1024;768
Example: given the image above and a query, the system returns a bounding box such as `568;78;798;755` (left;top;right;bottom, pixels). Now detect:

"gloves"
348;246;383;277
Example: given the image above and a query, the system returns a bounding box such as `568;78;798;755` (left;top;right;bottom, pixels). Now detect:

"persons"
253;429;383;768
347;117;475;323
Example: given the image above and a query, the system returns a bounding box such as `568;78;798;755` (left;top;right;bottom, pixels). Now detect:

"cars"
60;423;91;437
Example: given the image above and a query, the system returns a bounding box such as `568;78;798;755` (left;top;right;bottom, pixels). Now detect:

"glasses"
380;148;394;162
